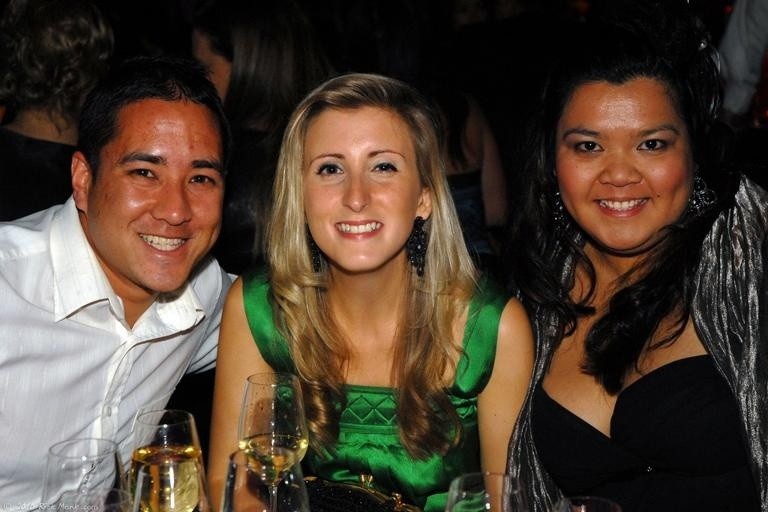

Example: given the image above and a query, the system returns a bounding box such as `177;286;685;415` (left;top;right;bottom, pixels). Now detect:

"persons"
203;73;535;511
489;0;768;512
2;1;768;279
1;50;239;510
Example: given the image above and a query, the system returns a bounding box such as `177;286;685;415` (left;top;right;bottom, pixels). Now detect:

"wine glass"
235;371;309;512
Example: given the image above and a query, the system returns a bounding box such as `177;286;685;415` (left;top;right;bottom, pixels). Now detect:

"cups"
221;448;307;512
127;410;204;512
40;440;124;510
